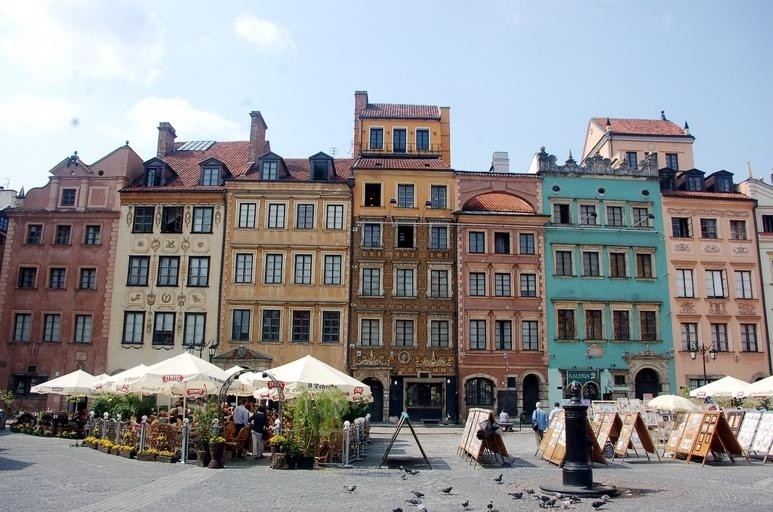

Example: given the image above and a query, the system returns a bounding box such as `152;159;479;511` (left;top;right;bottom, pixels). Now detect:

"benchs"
498;423;514;432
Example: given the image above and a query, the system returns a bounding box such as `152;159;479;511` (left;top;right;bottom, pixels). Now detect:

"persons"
229;397;251;460
267;411;280;460
703;396;713;404
499;407;512;431
549;403;561;426
248;406;269;460
532;401;548;447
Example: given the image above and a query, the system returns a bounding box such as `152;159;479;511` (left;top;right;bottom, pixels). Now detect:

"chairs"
224;421;251;462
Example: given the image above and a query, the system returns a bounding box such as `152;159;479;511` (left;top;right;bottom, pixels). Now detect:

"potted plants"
194;398;223;468
287;386;350;469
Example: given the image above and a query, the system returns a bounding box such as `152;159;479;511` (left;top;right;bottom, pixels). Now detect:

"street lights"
689;341;718;386
206;340;215;363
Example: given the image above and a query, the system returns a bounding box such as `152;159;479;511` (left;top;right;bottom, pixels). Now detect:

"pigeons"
398;465;420;480
508;492;523;499
392;489;427;512
440;486;452;494
493;474;503;484
478;419;506;437
525;487;534;493
343;484;357;493
502;458;516;468
461;500;469;509
486;500;494;512
592;494;611;508
534;491;581;510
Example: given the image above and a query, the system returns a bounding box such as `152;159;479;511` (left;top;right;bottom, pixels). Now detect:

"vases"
208;444;224;468
271;443;283;460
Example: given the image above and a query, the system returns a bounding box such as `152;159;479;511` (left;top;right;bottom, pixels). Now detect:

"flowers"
208;435;226;444
270;435;286;446
84;426;175;463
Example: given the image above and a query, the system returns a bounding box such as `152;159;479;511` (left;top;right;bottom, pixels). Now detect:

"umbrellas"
744;375;772;398
688;374;753;403
648;393;697;423
